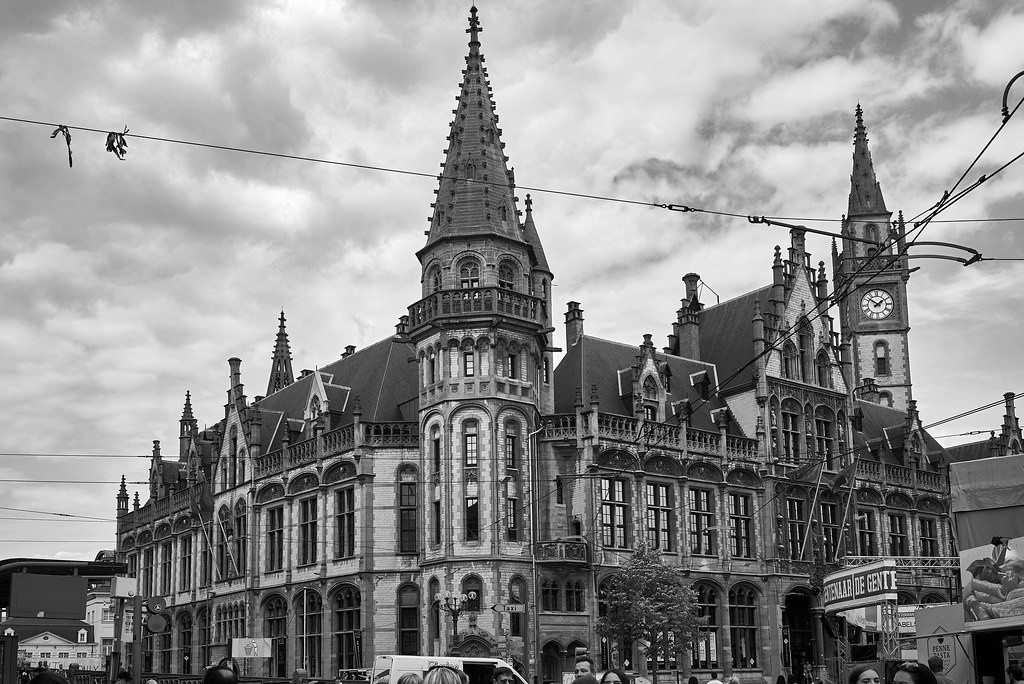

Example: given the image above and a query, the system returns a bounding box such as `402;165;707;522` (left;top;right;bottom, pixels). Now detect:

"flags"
200;480;214;519
187;486;200;517
828;460;859;493
784;456;823;484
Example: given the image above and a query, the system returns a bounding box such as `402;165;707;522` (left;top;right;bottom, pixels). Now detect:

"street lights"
433;591;478;657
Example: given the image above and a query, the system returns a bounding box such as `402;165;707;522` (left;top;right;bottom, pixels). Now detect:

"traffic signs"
491;604;526;614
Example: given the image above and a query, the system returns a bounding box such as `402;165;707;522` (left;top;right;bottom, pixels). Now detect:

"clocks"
860;289;894;320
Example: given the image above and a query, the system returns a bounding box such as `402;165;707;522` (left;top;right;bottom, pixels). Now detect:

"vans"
370;655;529;684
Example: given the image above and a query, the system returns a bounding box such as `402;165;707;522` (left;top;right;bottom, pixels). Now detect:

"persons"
114;662;132;684
688;671;740;684
146;666;238;684
846;654;956;684
571;656;630;684
776;674;797;684
396;665;470;684
19;661;71;684
492;667;514;684
804;661;815;684
